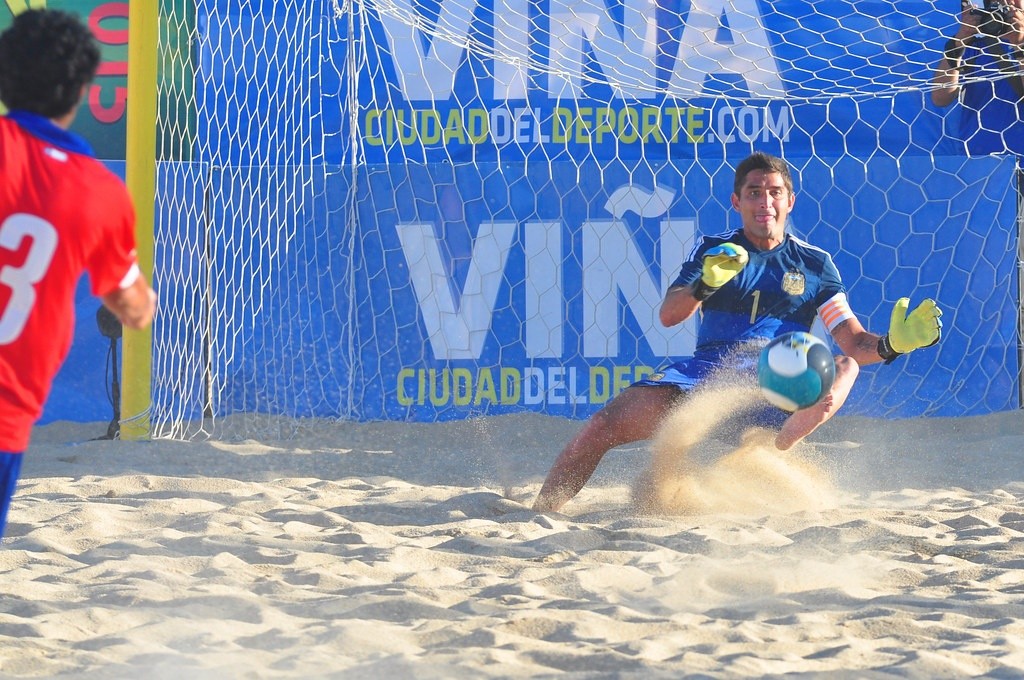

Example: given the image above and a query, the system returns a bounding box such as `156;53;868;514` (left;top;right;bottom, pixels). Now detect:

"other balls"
757;331;837;414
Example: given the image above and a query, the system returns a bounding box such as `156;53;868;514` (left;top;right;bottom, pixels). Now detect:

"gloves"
877;296;942;365
693;243;749;301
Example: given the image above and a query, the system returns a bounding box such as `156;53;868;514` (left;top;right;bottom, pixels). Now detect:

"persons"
530;151;943;508
932;0;1024;107
2;7;159;537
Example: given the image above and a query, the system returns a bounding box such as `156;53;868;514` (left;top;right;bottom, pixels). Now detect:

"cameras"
970;6;1011;37
